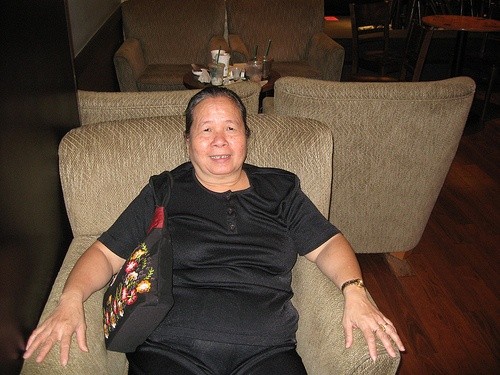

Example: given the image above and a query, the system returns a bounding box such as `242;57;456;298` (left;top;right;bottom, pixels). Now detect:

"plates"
192;69;209;76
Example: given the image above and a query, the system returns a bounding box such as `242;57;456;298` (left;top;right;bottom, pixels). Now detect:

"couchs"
228;0;345;83
262;76;477;257
76;80;261;127
22;113;402;375
115;0;228;93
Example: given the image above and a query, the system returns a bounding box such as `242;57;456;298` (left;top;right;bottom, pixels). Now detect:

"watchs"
341;279;368;296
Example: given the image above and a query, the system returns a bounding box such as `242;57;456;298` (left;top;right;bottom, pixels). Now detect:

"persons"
22;86;406;375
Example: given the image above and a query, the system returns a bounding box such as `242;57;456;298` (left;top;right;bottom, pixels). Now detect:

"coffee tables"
183;64;281;109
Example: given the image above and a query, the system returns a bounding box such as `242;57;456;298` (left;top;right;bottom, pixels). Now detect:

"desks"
398;14;500;123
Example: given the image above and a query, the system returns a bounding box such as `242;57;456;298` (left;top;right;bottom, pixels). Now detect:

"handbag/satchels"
104;170;175;353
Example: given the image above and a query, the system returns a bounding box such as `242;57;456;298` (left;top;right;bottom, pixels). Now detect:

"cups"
210;49;226;61
215;52;231;77
209;62;225;85
259;55;275;77
246;58;264;82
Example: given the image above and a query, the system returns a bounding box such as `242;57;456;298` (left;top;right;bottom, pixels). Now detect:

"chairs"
481;0;500;60
349;0;408;76
